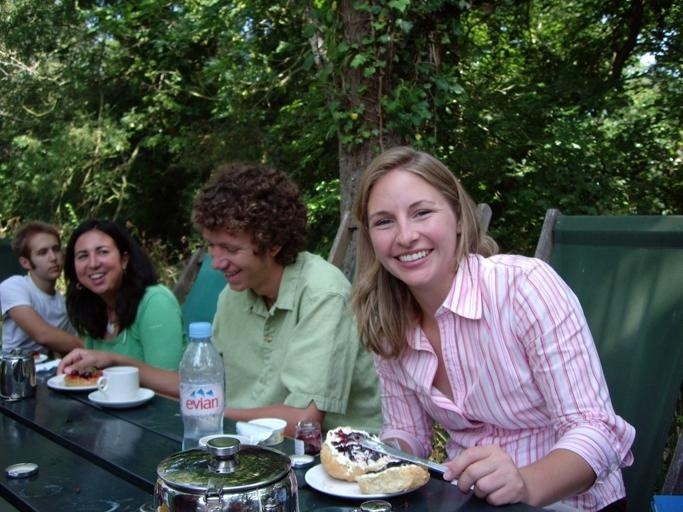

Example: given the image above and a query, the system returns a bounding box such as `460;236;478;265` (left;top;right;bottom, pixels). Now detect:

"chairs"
328;198;492;294
168;250;229;346
532;207;683;511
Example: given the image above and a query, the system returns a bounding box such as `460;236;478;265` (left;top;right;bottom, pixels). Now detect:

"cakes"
63;366;103;387
321;424;396;479
31;351;41;361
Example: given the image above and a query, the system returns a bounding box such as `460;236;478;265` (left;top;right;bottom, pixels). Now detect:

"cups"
234;417;287;446
97;366;139;401
198;434;246;447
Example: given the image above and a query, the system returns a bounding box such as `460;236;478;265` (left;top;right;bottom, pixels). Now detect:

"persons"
0;224;86;359
62;219;183;372
353;147;636;512
57;163;383;443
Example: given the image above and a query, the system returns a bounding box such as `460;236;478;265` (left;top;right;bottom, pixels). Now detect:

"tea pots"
0;347;35;402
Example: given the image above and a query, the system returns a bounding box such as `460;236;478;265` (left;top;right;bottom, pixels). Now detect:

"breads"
358;464;431;495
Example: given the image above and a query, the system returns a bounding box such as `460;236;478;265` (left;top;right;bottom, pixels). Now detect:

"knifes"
357;436;463;479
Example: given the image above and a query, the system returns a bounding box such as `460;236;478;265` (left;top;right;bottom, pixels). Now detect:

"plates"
304;458;430;499
33;353;49;364
88;388;154;407
46;373;102;390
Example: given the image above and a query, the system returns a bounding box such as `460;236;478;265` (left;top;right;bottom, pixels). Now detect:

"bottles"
180;323;225;452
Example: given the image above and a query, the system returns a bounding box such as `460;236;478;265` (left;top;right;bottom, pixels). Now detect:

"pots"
154;436;299;512
